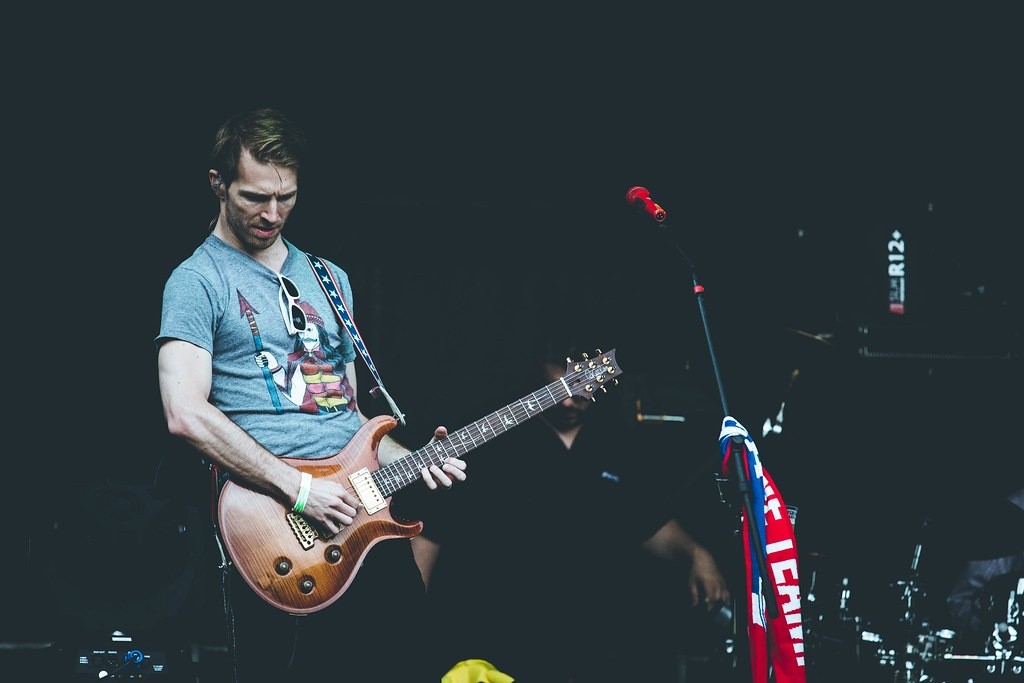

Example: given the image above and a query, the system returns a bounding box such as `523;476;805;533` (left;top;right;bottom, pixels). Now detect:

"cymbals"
785;313;857;364
612;388;690;427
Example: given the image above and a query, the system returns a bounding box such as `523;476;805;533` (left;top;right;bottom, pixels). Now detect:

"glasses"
276;273;308;338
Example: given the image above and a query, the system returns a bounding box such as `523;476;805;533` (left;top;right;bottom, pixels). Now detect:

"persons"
153;107;467;683
407;341;734;682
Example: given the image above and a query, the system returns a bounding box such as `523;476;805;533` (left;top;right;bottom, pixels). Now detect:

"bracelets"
292;472;312;515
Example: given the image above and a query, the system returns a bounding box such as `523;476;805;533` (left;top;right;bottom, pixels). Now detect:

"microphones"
626;186;666;221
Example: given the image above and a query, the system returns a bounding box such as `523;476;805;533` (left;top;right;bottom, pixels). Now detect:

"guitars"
210;342;624;618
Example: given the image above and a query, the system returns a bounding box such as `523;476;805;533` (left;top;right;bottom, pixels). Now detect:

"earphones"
214;180;221;189
